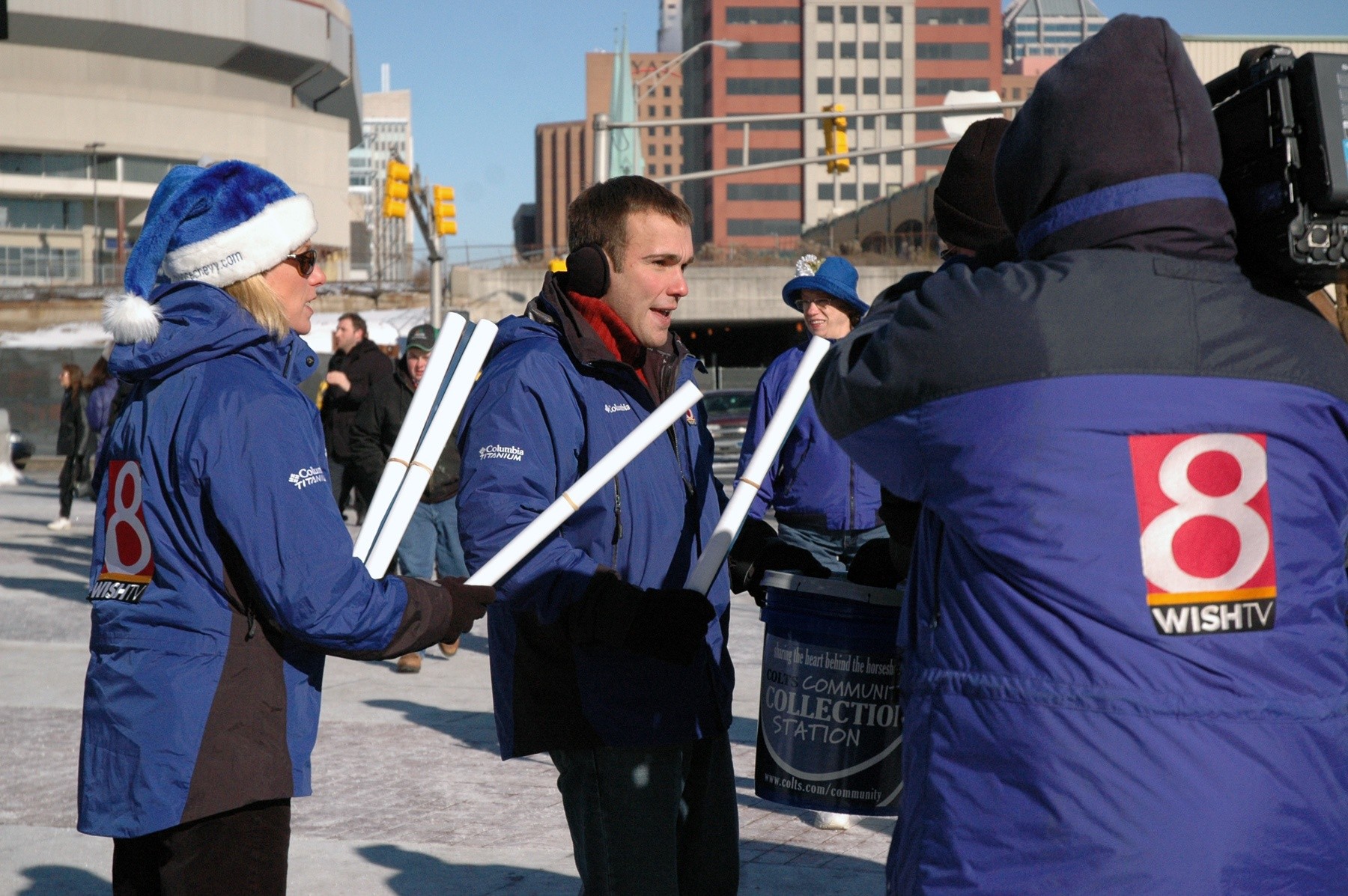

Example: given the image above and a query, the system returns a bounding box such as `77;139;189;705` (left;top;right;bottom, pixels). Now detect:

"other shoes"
815;810;865;829
397;651;420;673
438;636;459;657
48;517;68;531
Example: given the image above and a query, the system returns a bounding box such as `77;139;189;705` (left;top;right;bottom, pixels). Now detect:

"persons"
47;313;470;673
732;12;1348;896
458;175;832;896
76;160;496;896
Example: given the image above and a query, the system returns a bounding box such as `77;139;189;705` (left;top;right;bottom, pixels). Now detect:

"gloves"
846;539;902;590
625;589;718;660
753;538;829;603
433;574;497;646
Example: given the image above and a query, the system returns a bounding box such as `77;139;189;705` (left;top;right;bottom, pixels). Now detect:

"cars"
700;388;761;455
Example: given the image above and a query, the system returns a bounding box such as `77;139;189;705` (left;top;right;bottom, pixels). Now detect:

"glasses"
793;297;837;312
286;250;316;278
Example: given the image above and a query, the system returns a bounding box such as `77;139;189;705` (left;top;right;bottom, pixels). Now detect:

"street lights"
633;39;742;176
86;141;105;290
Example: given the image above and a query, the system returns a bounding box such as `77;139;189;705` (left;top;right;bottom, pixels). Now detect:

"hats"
403;325;438;352
104;159;317;347
934;117;1013;250
781;255;872;316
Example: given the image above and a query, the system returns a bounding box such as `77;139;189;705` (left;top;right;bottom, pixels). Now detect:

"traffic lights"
433;183;457;237
383;159;410;218
